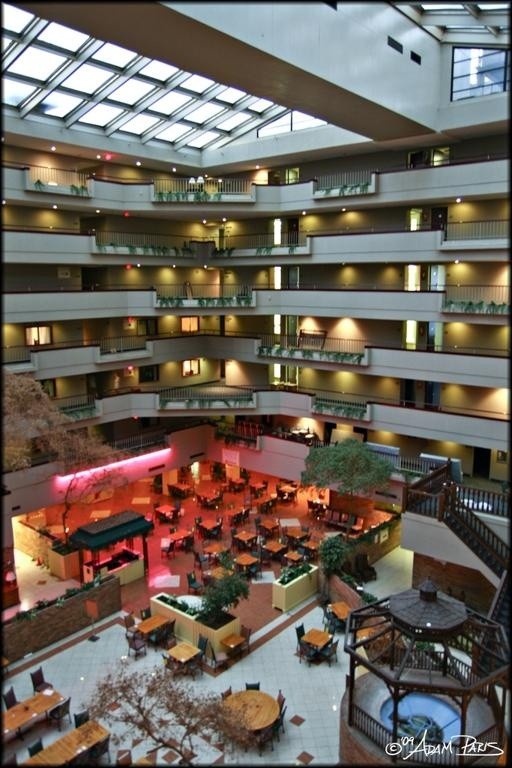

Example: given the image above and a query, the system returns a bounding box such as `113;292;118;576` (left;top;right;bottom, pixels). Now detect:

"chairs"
125;633;146;661
278;689;285;711
258;526;266;537
161;540;176;560
185;655;203;681
140;605;151;619
338;512;347;527
196;494;213;510
220;685;232;699
288;488;299;503
246;565;262;580
198;525;207;539
209;641;229;673
283;526;290;544
3;754;17;767
161;652;184;681
73;709;93;727
207;552;217;569
330;512;340;525
302;526;309;532
182;479;195;496
187;570;202;595
250;485;259;497
246;536;258;551
168;510;179;523
218;517;223;538
2;686;20;710
352;518;363;533
287;483;297;501
167;638;177;663
345;512;354;533
195;516;201;533
170;528;176;534
261;548;271;568
299;643;314;668
276;483;286;501
175;501;180;517
193;550;206;569
155;617;176;646
255;517;262;534
321;510;331;525
213;488;223;506
181;536;191;554
236;625;251;659
198;634;208;663
27;738;43;756
124;610;144;640
295;623;306;654
246;681;260;690
256;725;275;755
30;666;53;696
263;479;269;495
144;510;154;528
274;706;291;742
16;725;34;740
232;536;244;553
322;607;335;625
168;484;186;498
153;503;160;511
48;697;72;731
318;639;340;667
324;624;336;643
93;737;110;766
271;526;280;537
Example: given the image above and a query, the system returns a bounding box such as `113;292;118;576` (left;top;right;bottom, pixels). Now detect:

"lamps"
188;177;195;183
197;176;205;183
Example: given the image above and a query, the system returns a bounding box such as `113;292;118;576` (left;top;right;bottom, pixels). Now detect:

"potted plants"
317;535;346;576
